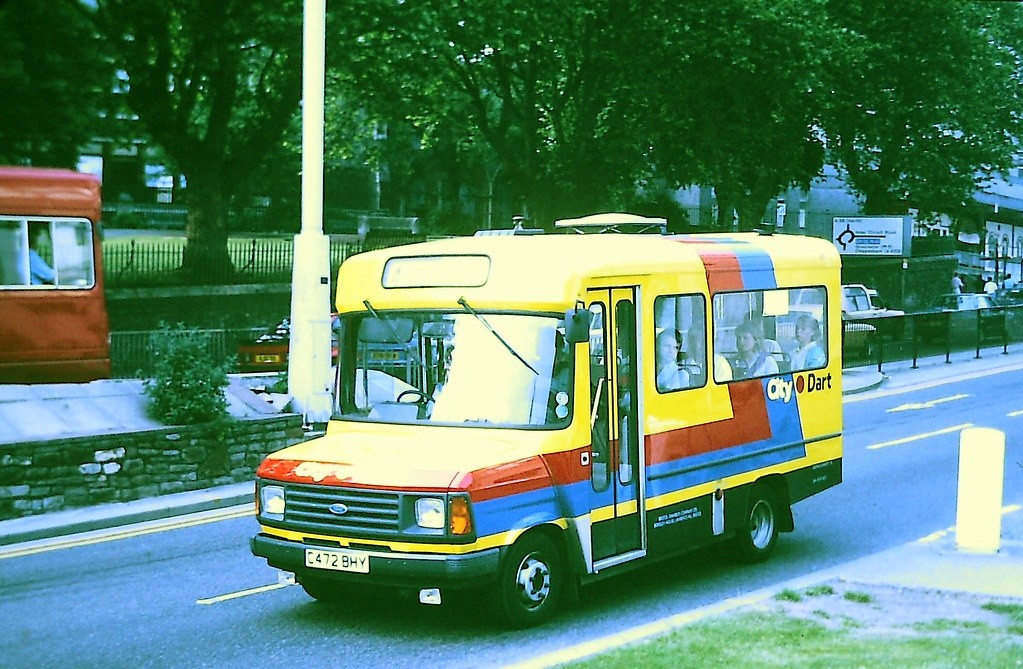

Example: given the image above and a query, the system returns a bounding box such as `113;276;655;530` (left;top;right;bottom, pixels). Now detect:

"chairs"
590;334;794;398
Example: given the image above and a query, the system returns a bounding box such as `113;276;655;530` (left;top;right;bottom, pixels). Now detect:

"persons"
951;272;965;294
15;225;85;286
1004;273;1015;289
423;306;830;425
983;276;998;295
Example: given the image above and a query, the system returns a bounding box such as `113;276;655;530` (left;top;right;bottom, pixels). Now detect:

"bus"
0;164;110;382
251;229;846;632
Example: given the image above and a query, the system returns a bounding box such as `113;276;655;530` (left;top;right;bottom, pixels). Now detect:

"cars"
916;292;998;322
242;308;615;371
1000;286;1023;308
779;284;904;334
